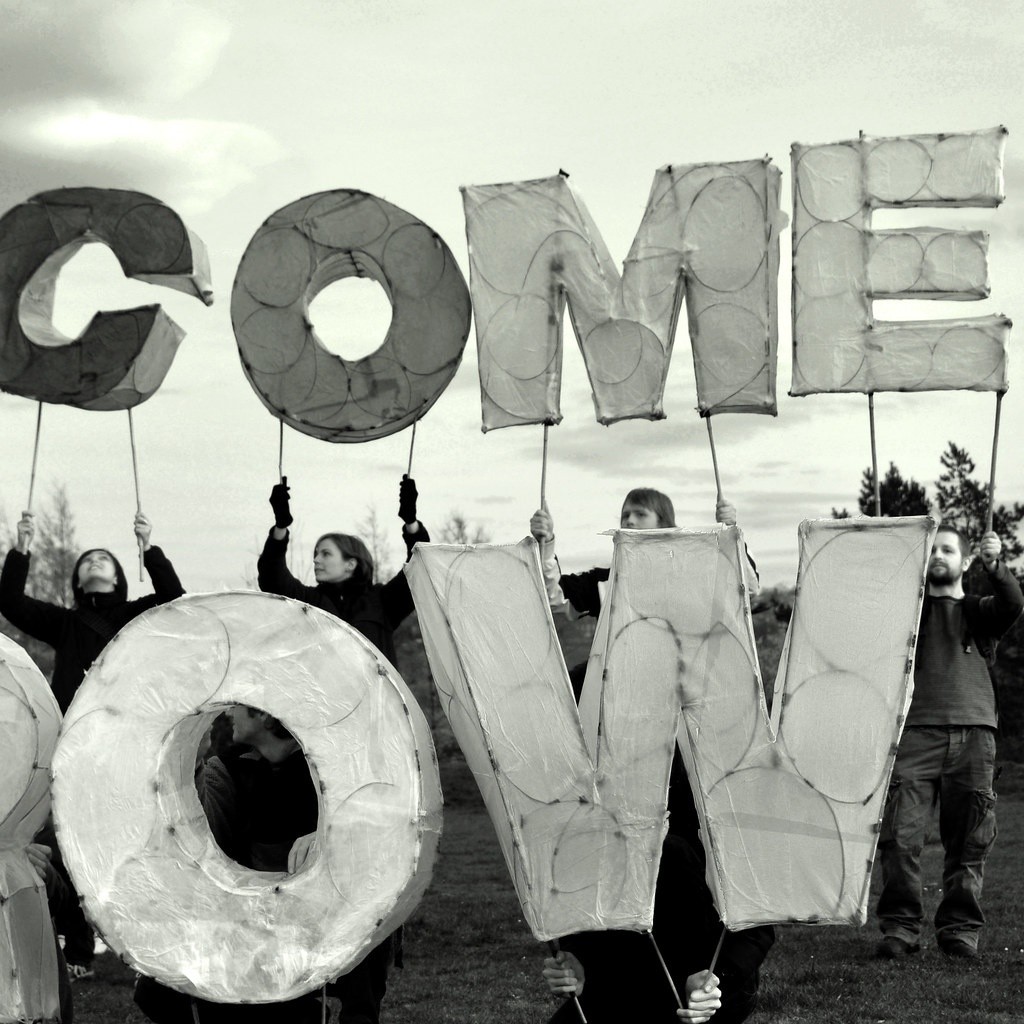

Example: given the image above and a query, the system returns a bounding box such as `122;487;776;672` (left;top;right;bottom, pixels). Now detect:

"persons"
1;511;192;966
530;490;757;710
536;744;773;1022
255;474;433;687
872;530;1023;963
193;702;390;1022
20;814;98;1022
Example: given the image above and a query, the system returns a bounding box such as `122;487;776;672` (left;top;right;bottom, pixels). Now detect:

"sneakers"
942;940;982;962
877;936;921;956
64;964;96;983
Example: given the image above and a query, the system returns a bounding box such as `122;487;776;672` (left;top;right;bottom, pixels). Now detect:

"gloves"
269;475;294;529
398;473;418;524
